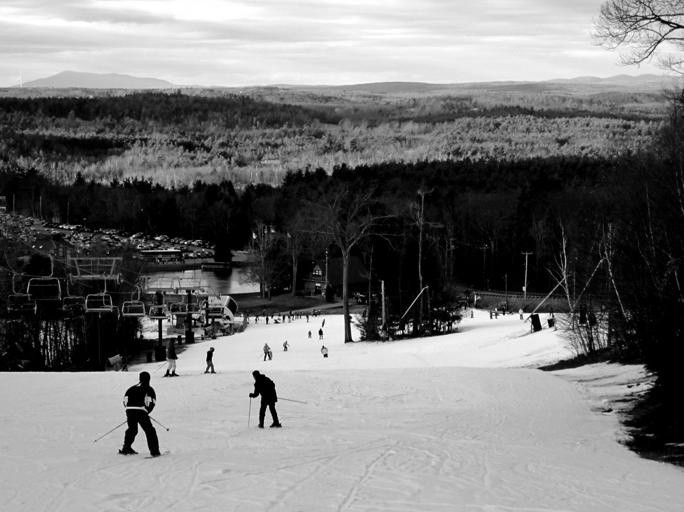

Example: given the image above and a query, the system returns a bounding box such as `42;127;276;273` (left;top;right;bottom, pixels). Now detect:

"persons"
118;371;160;457
246;308;325;327
249;370;281;429
319;328;324;340
320;345;328;358
204;347;216;374
163;337;180;376
263;343;271;361
519;308;523;319
282;340;289;352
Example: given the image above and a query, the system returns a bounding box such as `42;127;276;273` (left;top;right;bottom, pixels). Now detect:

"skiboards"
118;448;170;459
